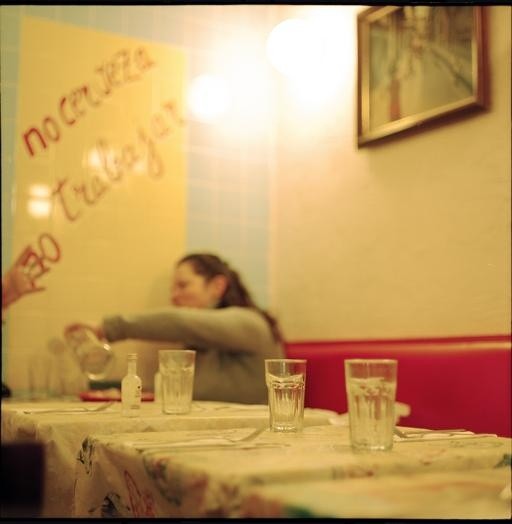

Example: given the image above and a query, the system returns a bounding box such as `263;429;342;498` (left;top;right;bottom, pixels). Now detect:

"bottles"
63;321;116;384
118;351;143;415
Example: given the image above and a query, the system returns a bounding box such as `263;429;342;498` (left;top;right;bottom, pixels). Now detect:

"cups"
343;355;399;451
156;347;200;413
263;357;312;435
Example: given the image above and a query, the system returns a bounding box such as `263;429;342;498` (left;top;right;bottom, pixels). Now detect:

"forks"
19;399;114;414
392;426;466;439
131;423;272;448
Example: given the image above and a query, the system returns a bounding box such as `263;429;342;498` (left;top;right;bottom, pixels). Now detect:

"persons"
66;251;290;405
386;66;402;121
1;242;52;313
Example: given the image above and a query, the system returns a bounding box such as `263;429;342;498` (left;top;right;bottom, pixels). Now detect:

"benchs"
281;331;512;436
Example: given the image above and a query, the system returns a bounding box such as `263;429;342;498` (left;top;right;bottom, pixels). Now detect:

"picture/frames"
358;7;490;149
